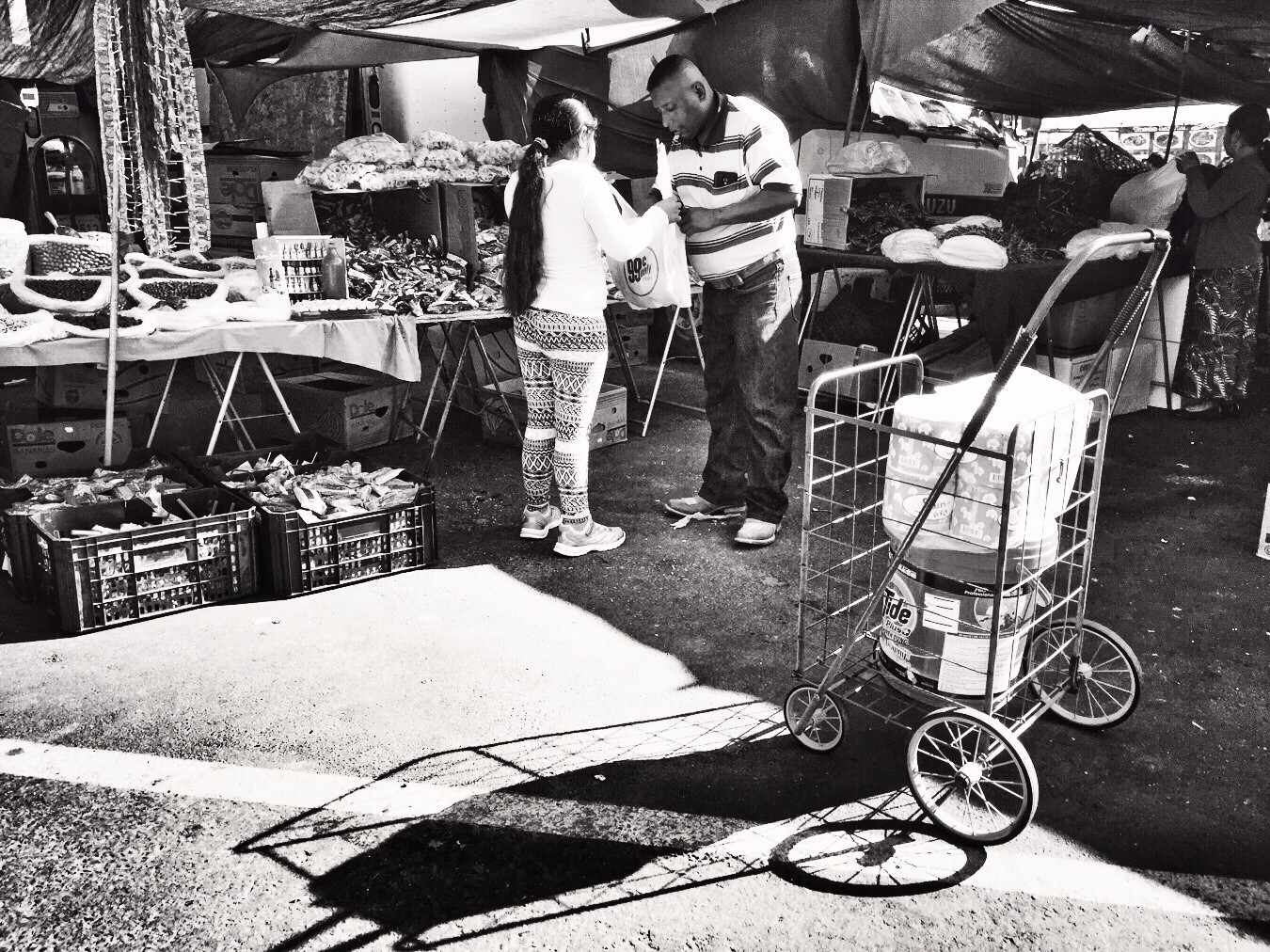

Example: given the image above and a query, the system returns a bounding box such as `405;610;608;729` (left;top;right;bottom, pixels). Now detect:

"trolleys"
786;224;1175;850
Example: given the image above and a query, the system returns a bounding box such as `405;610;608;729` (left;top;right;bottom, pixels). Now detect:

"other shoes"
1174;402;1242;420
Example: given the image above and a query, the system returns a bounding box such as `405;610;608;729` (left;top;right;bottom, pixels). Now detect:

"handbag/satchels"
607;184;694;314
1110;148;1196;230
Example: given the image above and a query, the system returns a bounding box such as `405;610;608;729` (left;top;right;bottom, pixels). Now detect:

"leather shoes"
664;494;747;518
735;518;782;546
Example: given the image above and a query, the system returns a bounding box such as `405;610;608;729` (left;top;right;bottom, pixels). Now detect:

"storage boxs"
796;337;892;401
208;203;268;237
0;428;442;635
441;179;509;273
252;235;335;294
606;324;649;368
35;359;176;416
479;376;629;457
262;180;444;258
1;402;137;484
921;337;999;395
605;301;655;328
1031;338;1157;423
260;371;421;452
427;320;523;414
205;150;312;204
316;355;364;374
1039;285;1141;352
196;348;315;393
803;169;932;252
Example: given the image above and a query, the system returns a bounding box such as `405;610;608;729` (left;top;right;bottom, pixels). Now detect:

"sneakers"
521;504;562;540
554;516;626;557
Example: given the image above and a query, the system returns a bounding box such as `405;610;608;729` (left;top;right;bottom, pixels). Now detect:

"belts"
710;247;789;290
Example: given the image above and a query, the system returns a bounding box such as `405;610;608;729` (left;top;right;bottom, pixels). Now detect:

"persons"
492;89;686;559
1162;102;1270;420
641;50;809;547
